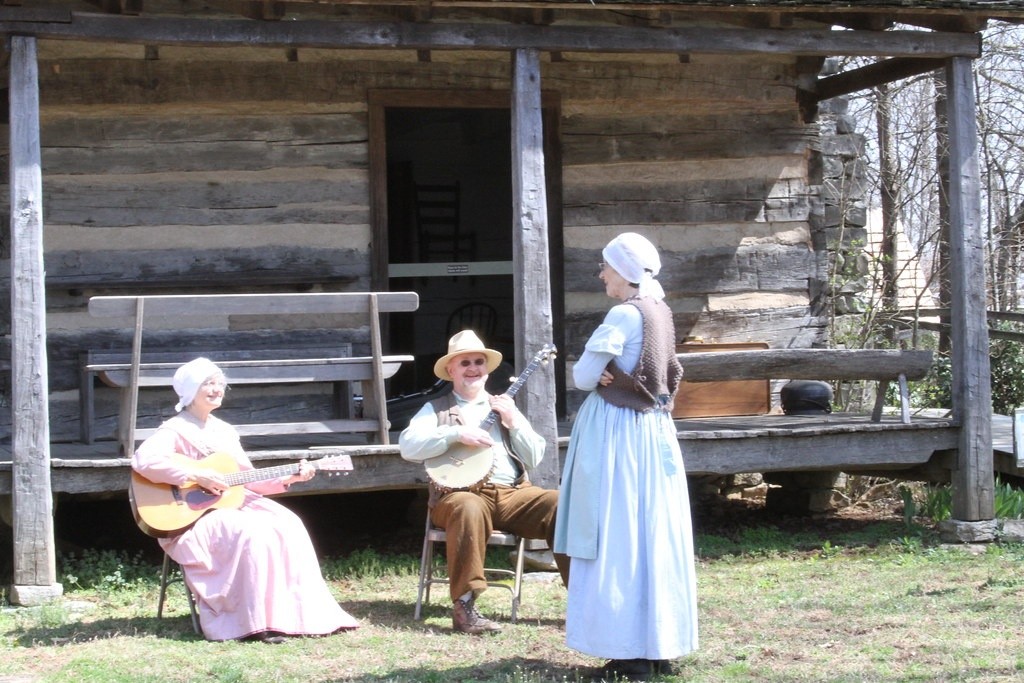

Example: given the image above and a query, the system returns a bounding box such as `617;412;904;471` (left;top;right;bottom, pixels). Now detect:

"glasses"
459;358;484;367
200;378;228;388
599;262;611;271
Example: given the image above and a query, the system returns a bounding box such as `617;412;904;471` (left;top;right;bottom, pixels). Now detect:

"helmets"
780;381;834;415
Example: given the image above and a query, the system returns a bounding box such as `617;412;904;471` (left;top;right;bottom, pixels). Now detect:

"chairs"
156;553;204;638
412;495;530;626
444;301;500;349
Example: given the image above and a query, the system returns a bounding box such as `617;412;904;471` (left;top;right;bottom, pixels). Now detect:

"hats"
174;357;222;411
432;329;503;381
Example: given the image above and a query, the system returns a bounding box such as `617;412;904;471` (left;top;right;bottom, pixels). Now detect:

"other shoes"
586;659;653;680
651;659;674;675
248;632;286;644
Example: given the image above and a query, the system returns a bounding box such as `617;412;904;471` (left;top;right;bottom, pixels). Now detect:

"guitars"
128;455;353;539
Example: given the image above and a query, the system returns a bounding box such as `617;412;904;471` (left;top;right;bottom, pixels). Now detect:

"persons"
398;330;572;634
130;357;362;643
552;234;701;683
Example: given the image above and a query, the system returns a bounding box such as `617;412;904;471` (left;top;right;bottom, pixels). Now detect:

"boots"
452;598;501;634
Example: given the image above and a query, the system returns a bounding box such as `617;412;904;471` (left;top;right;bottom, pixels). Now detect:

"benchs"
675;345;934;428
83;291;422;458
